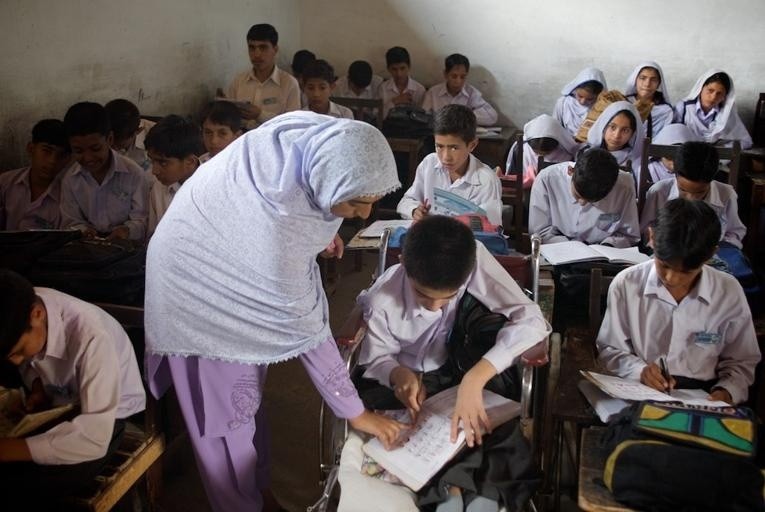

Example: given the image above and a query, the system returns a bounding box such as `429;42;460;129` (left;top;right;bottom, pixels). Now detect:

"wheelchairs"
306;227;552;512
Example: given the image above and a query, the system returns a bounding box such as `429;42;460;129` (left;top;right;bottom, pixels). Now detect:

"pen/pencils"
422;199;428;214
417;372;424;401
19;386;27;408
659;357;671;396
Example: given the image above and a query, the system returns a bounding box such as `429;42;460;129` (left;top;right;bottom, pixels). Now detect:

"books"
0;402;77;439
361;384;528;493
575;368;737;422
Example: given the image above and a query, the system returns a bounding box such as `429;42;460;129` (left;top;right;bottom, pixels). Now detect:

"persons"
139;105;409;512
507;60;764;308
225;24;301;130
293;46;499;126
353;213;552;448
397;104;503;230
594;198;761;415
2;99;242;239
0;272;148;471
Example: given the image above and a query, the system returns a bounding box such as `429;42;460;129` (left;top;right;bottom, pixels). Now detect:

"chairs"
87;299;174;512
